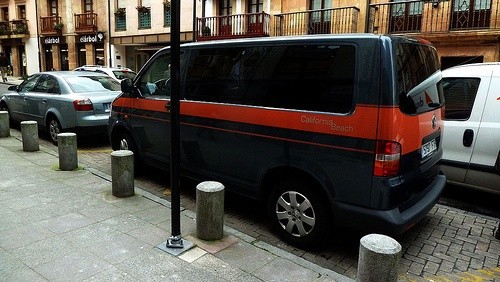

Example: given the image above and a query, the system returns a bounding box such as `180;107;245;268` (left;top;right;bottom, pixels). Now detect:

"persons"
0;52;9;82
22;55;27;76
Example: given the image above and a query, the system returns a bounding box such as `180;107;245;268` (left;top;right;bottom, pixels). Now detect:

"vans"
441;62;500;195
107;32;449;252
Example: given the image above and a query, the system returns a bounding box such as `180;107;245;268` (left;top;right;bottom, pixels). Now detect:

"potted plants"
162;0;170;10
53;22;64;33
114;8;126;20
137;6;149;16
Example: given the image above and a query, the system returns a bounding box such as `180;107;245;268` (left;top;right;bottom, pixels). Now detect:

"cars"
73;65;137;85
0;71;119;146
